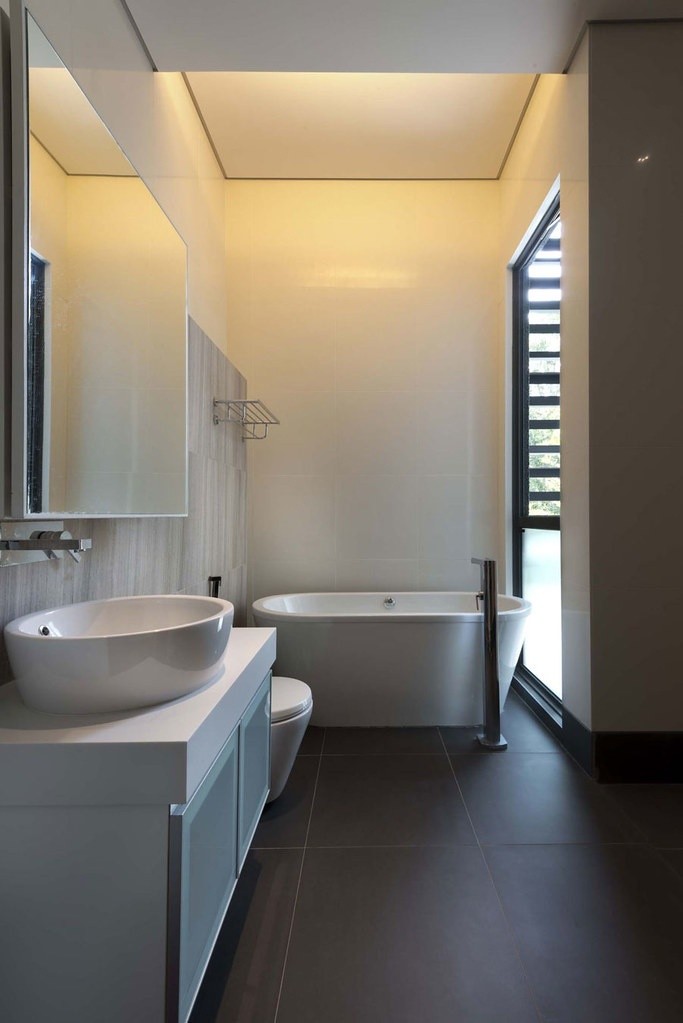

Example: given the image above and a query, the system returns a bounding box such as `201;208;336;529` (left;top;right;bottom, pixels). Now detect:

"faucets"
8;530;93;564
470;557;509;750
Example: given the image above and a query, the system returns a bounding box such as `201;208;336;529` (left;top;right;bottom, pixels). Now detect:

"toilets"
267;675;314;804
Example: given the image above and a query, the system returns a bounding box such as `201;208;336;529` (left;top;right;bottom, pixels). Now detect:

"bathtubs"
250;591;533;728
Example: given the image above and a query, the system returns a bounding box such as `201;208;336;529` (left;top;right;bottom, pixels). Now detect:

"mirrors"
10;0;188;524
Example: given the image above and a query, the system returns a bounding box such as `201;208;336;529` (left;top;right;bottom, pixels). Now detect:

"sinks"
4;593;235;715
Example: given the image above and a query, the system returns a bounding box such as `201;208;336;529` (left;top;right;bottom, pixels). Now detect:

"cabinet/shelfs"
0;666;281;1023
